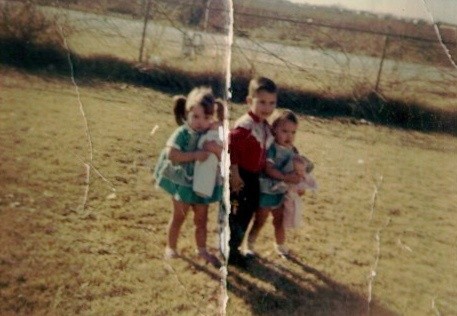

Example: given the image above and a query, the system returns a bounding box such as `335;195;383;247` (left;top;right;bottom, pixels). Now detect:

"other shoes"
164;250;178;258
275;245;289;257
245;249;254;258
228;250;253;269
195;252;216;262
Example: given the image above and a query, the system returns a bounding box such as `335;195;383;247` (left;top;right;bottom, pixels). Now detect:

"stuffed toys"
194;119;223;198
270;154;315;197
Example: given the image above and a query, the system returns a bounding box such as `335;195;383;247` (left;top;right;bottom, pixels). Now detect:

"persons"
238;108;303;264
227;75;277;265
156;86;231;264
